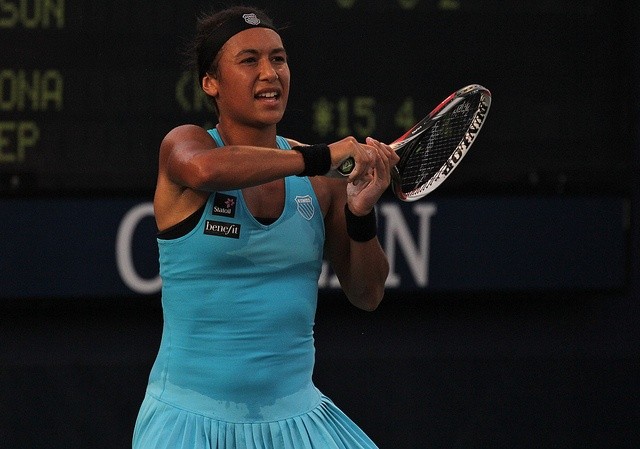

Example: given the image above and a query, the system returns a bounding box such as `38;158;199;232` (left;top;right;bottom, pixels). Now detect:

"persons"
131;0;402;449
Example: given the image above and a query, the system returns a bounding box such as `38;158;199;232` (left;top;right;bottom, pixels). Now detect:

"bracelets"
342;202;378;243
290;144;332;179
